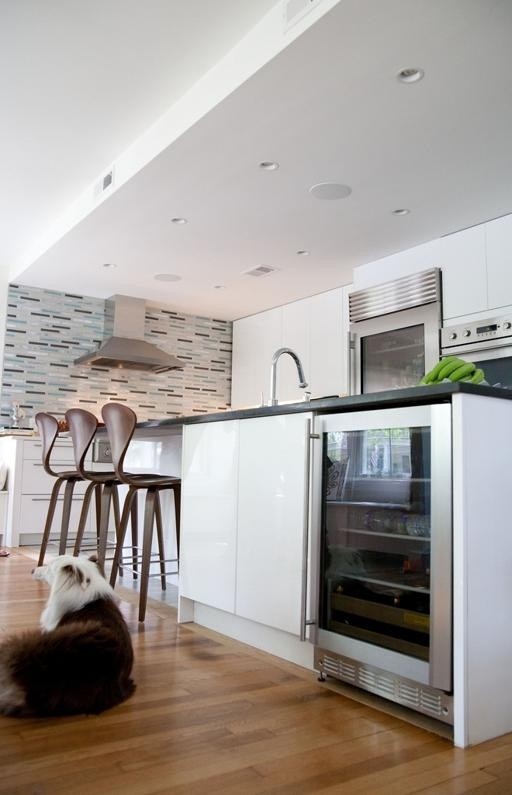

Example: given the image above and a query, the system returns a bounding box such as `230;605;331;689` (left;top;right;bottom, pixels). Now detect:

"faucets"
268;346;308;407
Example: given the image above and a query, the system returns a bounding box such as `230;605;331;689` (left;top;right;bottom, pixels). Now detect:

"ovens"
440;335;512;390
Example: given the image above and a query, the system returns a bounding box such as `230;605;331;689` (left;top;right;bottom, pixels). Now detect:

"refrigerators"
346;268;443;393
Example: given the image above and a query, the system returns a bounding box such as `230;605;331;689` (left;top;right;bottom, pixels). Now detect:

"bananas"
419;355;485;387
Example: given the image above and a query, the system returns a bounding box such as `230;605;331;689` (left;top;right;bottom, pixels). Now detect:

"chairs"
33;402;180;623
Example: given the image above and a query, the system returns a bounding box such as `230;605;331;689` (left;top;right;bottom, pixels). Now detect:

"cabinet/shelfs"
0;435;91;548
181;412;316;642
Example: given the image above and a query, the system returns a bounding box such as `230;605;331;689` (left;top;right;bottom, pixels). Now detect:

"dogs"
0;556;138;720
